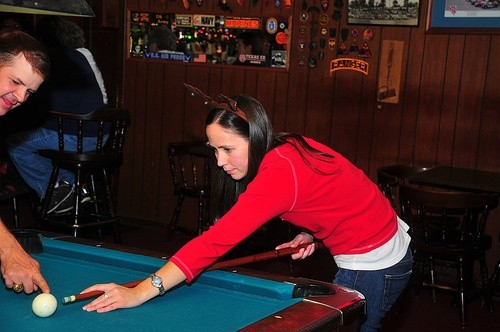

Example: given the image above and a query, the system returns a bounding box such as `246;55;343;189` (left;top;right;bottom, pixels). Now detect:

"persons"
79;94;411;332
0;19;112;292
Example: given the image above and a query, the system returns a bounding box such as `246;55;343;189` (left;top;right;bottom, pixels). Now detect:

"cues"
64;241;318;303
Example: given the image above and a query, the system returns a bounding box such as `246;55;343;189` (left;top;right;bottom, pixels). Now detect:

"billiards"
32;293;58;318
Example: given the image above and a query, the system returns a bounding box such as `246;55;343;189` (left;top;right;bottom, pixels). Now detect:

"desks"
0;229;367;332
377;165;500;312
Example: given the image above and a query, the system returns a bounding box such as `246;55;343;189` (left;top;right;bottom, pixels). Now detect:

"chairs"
165;141;212;243
378;171;495;329
37;108;132;244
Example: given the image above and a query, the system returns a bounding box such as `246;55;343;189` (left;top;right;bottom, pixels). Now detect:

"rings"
13;284;23;292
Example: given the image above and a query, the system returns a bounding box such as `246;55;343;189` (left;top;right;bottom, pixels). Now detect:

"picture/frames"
125;7;293;73
425;0;500;35
346;0;422;28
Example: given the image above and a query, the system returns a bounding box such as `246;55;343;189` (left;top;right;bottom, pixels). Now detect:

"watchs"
149;273;165;295
